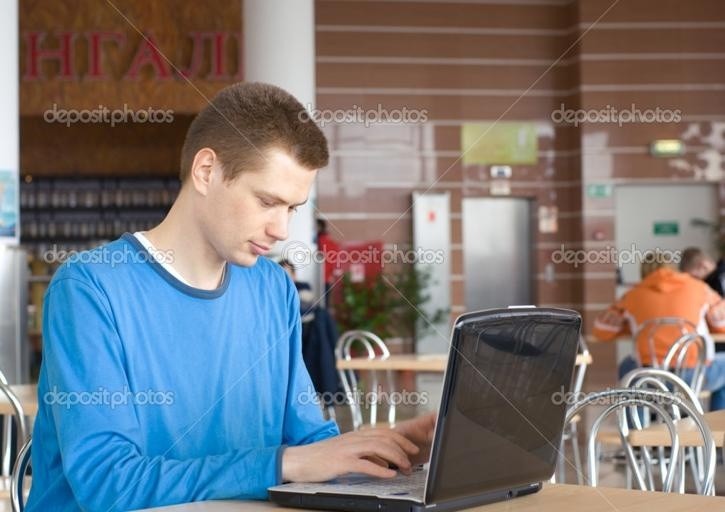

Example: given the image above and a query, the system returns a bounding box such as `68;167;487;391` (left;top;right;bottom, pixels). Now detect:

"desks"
125;480;725;512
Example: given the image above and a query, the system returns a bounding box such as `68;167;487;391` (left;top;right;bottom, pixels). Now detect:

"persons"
680;246;725;297
317;217;339;310
279;259;316;347
592;249;724;427
24;81;441;511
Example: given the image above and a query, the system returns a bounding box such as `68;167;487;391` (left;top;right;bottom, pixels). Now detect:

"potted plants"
340;264;447;405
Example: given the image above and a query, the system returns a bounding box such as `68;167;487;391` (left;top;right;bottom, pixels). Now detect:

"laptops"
266;306;583;512
